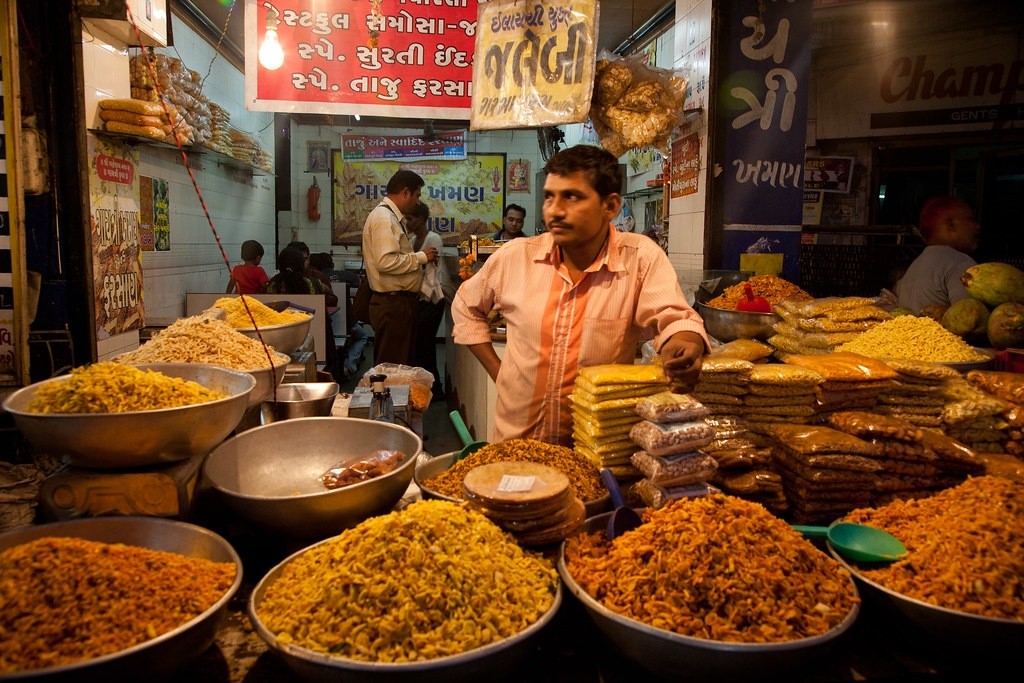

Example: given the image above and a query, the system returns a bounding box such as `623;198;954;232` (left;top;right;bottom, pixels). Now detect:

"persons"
486;204;528;240
406;201;446;398
895;194;981;313
362;170;438;367
225;240;369;384
450;145;712;450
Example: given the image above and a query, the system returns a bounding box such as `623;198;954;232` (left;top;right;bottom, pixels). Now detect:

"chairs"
28;323;76;384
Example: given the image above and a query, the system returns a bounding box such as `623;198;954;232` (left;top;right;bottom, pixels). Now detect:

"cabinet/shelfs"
81;0;167;51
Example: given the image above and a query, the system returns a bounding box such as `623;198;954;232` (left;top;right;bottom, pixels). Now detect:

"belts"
374;292;420;298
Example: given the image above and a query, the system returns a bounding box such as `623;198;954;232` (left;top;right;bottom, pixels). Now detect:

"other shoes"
343;366;356;381
430;390;445;402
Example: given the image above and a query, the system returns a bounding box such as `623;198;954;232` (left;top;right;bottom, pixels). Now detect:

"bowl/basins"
826;512;1024;644
944;348;997;373
248;524;563;682
202;416;423;540
2;364;257;470
1;517;242;682
557;507;860;680
697;297;784;344
237;312;316;356
260;382;340;423
237;352;290;412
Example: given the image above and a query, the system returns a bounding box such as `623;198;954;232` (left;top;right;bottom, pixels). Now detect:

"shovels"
736;284;771;313
266;301;315;315
792;522;908;561
601;469;643;543
449;410;489;461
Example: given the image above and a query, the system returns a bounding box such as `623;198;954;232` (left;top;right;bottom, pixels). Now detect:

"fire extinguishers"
307;176;321;220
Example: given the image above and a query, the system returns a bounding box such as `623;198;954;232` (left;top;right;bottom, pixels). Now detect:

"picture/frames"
307;141;331;173
331;149;507;247
506;159;531;193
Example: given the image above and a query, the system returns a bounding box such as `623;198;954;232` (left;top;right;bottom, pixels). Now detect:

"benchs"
185;282;351;381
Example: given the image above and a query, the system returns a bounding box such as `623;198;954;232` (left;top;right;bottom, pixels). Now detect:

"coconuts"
887;261;1024;350
